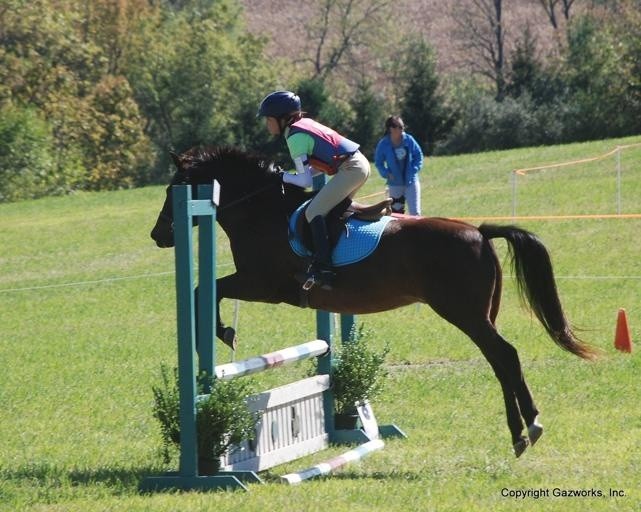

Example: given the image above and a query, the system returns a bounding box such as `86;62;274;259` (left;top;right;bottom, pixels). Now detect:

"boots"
303;214;337;279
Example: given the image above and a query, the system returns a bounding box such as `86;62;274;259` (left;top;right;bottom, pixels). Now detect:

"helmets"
256;90;301;119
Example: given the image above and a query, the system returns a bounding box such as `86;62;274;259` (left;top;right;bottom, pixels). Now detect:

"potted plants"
148;359;267;475
300;320;393;430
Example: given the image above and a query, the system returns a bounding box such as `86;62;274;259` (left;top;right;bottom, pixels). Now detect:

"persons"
254;90;372;291
375;115;424;215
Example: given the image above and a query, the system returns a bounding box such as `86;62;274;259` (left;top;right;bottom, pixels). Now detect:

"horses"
150;142;603;458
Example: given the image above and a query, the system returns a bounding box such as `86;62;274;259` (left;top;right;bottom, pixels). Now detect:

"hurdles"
139;170;409;493
